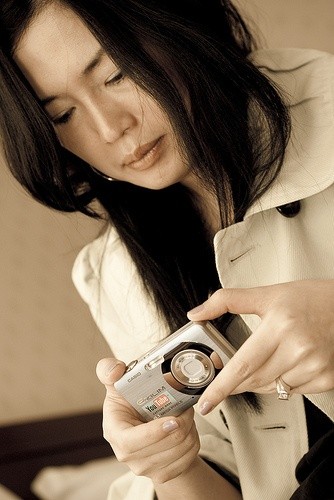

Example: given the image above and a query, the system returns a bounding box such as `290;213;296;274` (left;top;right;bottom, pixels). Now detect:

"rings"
277;377;293;401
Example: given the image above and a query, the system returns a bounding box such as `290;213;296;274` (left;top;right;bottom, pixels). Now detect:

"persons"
0;0;334;500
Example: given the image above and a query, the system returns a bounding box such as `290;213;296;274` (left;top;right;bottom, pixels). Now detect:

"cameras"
113;319;240;424
